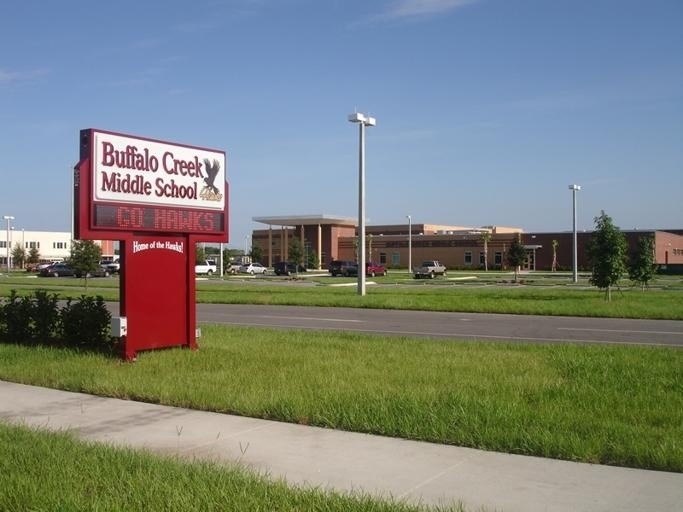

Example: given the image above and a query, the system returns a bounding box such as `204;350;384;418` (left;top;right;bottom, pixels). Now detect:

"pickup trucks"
413;260;446;279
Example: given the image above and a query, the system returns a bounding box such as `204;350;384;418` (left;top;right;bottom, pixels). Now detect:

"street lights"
348;112;376;296
243;234;249;256
569;184;582;283
3;216;25;272
405;215;412;273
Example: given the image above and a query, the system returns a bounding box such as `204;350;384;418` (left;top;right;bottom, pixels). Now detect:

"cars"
27;255;120;277
328;260;358;277
366;260;387;277
227;262;307;276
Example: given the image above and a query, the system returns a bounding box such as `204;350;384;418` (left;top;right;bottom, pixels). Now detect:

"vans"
195;259;216;276
